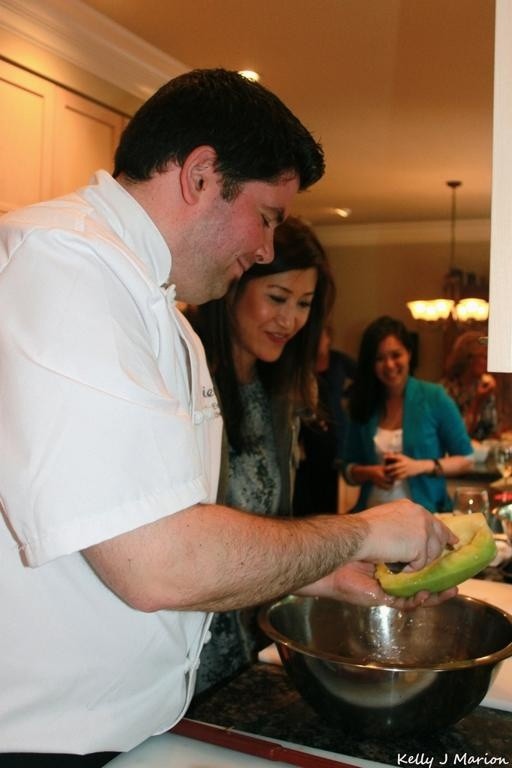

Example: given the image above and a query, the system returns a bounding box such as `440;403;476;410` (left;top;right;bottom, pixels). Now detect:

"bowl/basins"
257;591;511;731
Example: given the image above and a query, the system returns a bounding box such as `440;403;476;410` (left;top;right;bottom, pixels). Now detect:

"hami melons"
375;514;497;598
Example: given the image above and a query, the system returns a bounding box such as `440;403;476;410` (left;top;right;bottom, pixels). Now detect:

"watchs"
432;456;444;481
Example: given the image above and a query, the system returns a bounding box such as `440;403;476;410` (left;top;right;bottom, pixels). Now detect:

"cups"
451;482;490;523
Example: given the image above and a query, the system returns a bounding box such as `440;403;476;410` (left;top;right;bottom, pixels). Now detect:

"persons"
0;62;460;768
440;330;498;442
337;309;478;518
177;212;337;704
289;322;358;517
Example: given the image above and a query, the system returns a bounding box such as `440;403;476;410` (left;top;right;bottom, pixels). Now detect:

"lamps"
405;181;490;321
